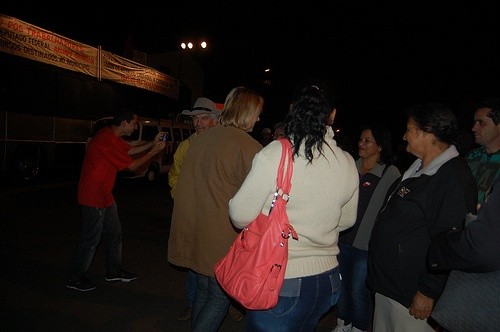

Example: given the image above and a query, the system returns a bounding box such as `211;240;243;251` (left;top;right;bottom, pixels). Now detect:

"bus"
86;116;196;181
176;113;195;124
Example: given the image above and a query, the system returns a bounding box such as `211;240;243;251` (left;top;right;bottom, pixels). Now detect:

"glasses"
360;137;375;143
272;133;284;137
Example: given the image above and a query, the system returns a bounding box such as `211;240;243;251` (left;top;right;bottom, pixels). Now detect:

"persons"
425;175;500;332
463;100;500;212
228;78;360;332
365;103;478;332
249;122;285;147
66;109;168;297
330;122;401;332
168;86;264;332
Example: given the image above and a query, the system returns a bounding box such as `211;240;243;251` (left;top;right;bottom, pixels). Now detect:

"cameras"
160;132;168;142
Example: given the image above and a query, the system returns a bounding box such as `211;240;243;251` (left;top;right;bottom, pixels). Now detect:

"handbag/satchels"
213;138;299;310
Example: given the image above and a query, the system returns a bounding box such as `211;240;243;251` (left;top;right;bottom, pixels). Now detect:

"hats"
263;128;271;134
182;98;222;119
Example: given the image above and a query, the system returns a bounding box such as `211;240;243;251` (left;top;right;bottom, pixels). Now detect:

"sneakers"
66;280;96;291
105;271;136;282
